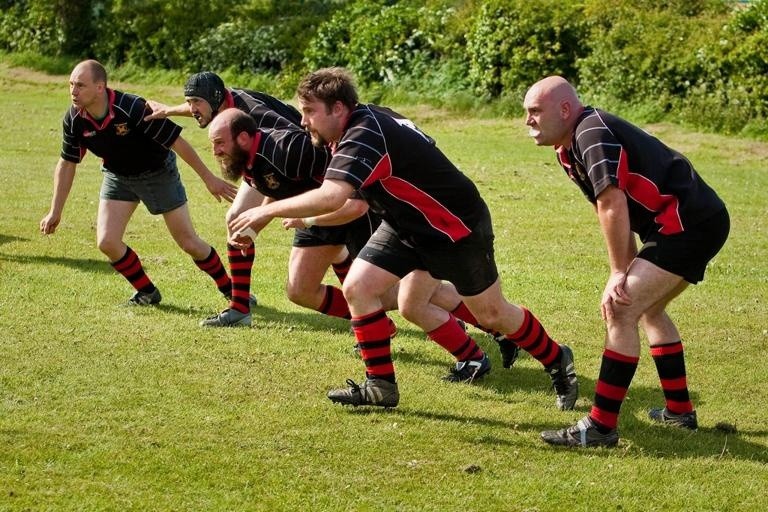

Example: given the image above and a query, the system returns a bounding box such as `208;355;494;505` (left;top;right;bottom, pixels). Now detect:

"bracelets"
302;216;316;228
241;228;257;240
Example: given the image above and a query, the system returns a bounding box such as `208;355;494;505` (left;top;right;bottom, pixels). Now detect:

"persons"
143;70;354;329
227;65;579;413
522;74;731;449
39;59;259;311
208;106;403;350
283;140;524;382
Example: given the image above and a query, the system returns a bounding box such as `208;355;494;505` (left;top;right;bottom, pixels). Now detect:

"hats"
183;71;227;113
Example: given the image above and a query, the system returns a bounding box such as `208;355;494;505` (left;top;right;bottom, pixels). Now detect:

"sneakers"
199;293;259;330
439;350;493;385
326;376;400;408
540;414;620;448
647;407;699;430
492;328;522;370
117;286;162;308
544;342;579;412
